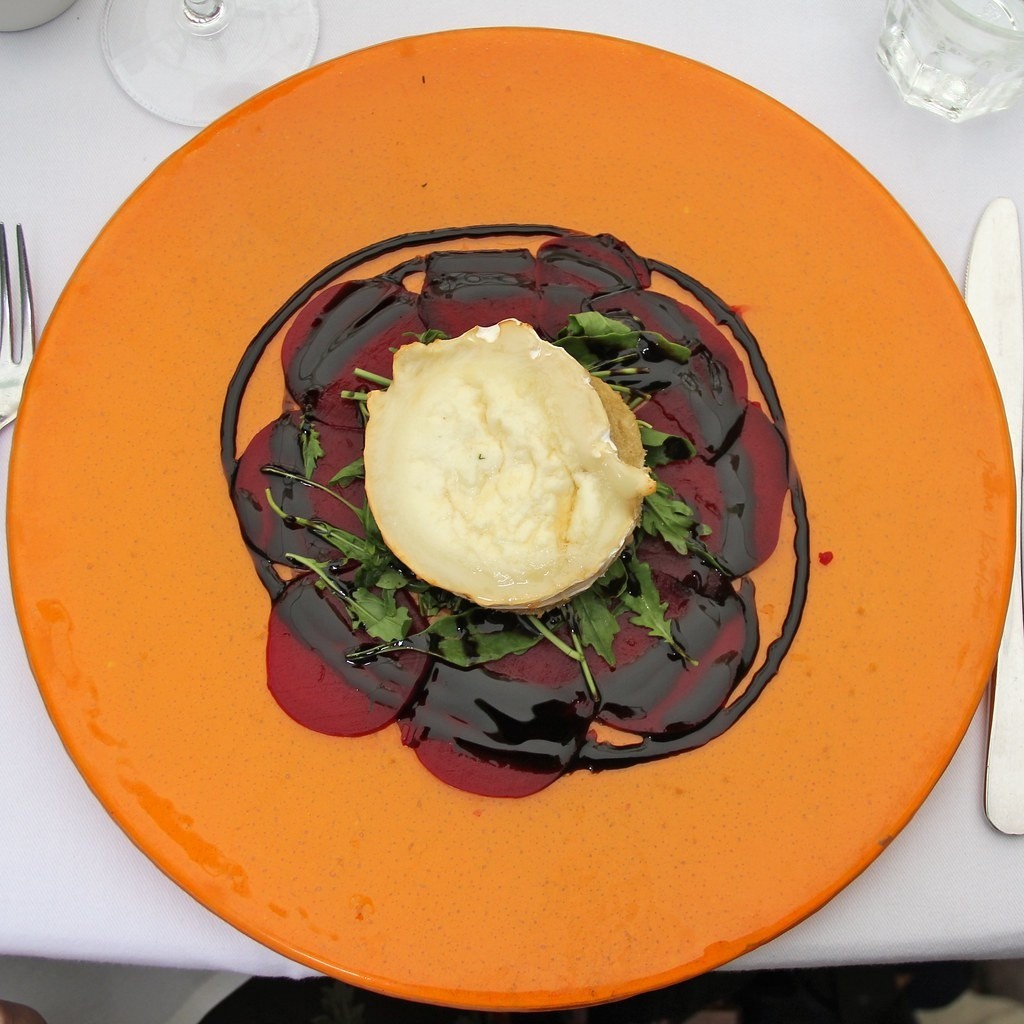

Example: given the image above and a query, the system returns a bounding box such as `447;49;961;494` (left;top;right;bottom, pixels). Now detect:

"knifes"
962;197;1024;835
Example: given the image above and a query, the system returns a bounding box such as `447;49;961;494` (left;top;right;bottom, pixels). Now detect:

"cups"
875;0;1024;124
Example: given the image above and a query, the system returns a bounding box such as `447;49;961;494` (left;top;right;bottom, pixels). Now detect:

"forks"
0;219;35;430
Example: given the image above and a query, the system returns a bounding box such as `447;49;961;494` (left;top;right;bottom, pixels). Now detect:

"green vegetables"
258;313;733;704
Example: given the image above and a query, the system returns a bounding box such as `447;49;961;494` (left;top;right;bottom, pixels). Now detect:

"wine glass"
98;0;321;128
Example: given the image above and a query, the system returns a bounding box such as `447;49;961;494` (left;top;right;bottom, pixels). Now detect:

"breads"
360;319;657;612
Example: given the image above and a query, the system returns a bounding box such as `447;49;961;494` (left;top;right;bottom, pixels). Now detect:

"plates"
5;23;1018;1014
